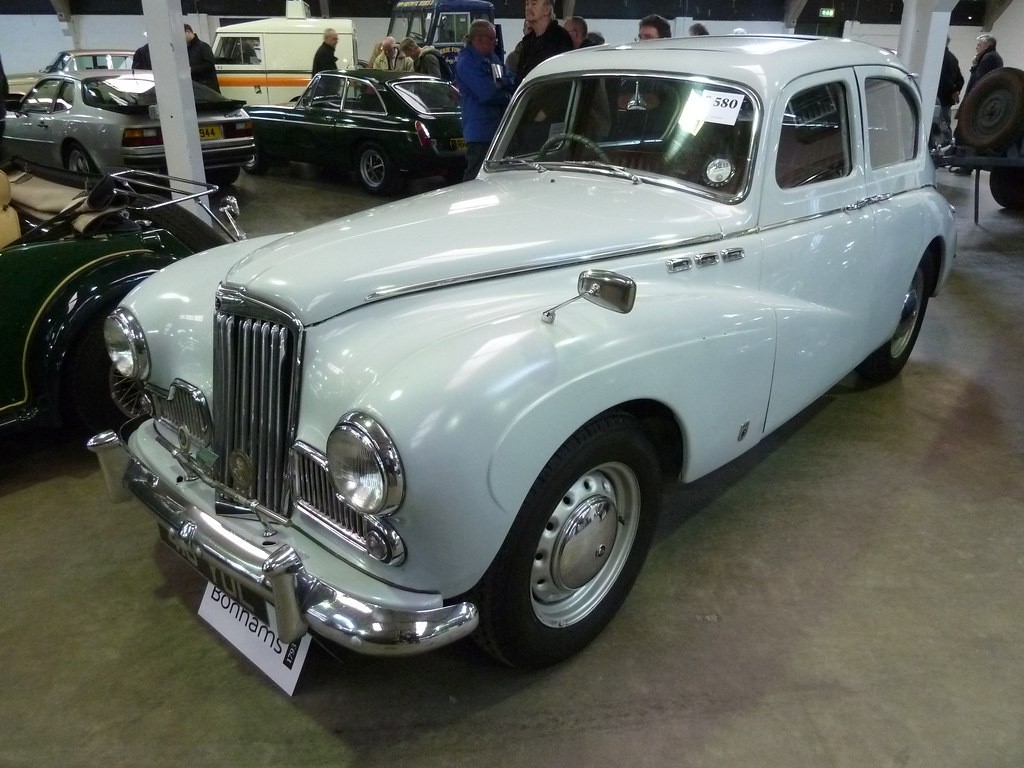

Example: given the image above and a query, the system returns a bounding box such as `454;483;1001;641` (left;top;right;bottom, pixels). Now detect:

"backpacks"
420;50;453;81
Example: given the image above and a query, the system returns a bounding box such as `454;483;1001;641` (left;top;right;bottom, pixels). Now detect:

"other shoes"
955;167;971;176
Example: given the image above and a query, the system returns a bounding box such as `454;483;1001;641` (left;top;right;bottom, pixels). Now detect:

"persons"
184;23;222;95
310;28;341;97
373;36;414;72
928;35;1004;176
132;43;152;70
0;55;9;142
455;20;511;182
399;37;453;81
505;0;710;151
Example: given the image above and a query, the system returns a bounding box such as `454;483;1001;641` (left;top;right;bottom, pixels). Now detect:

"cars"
209;1;500;112
85;33;963;698
237;66;476;197
0;65;257;184
35;48;138;76
0;169;248;460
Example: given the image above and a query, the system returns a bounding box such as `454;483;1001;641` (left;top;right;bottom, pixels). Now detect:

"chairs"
0;169;21;250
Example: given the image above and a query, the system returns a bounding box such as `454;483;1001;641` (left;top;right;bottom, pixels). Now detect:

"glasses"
482;34;498;44
